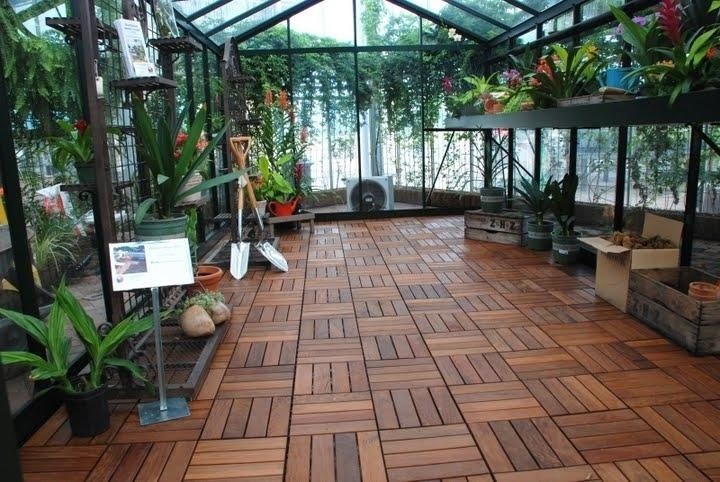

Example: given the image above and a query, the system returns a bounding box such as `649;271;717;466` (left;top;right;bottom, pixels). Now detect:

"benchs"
266;214;315;238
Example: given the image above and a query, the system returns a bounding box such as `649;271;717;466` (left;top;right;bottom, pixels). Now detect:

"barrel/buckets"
528;221;553;250
134;213;188;241
179;173;203;203
480;187;505;212
67;376;110;437
551;230;581;264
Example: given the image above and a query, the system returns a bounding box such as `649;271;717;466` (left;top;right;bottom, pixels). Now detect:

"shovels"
229;137;289;280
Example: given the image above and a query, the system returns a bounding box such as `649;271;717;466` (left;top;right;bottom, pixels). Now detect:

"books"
112;18;151;79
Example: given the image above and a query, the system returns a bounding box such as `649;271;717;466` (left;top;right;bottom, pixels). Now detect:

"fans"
347;175;394;212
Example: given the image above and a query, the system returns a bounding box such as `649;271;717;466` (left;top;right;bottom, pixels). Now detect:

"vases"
172;167;203;207
189;265;223;296
74;160;97;184
251;200;268;217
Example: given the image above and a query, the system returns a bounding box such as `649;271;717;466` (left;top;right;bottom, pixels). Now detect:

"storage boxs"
463;207;530;247
0;288;56;380
626;267;720;353
575;212;683;315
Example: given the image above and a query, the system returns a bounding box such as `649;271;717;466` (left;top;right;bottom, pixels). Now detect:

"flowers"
246;173;270;201
442;0;719;116
45;120;123;162
167;129;212;172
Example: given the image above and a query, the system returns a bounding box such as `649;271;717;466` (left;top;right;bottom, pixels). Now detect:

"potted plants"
110;91;255;241
548;198;581;264
457;135;517;212
0;271;177;436
510;175;561;250
